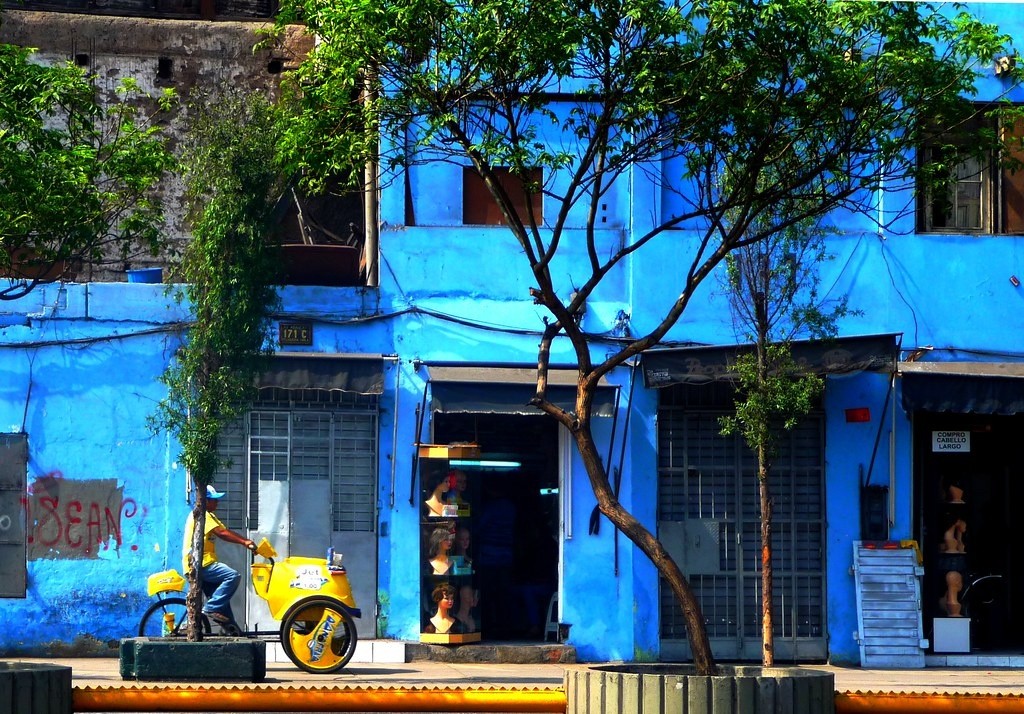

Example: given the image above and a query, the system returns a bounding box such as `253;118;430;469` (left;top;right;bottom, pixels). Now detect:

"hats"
194;485;226;499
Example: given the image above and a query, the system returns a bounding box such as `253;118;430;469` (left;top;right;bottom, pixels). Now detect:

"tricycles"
138;535;363;674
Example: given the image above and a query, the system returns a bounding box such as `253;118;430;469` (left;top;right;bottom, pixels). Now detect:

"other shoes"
201;611;229;623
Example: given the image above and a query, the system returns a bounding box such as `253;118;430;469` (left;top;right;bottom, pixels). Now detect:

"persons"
944;475;967;617
182;481;257;634
424;470;478;635
